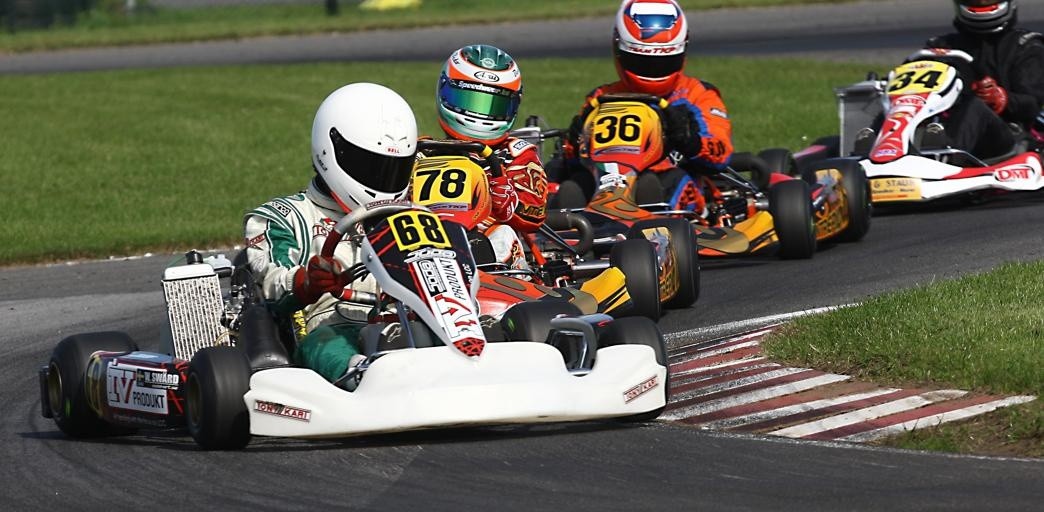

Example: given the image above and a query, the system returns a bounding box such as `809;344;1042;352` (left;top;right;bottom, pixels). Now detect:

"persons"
855;0;1044;167
548;0;734;209
240;82;498;390
406;44;548;281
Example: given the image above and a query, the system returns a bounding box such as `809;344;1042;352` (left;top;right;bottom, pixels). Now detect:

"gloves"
292;254;342;306
971;77;1008;116
487;163;519;222
664;103;702;158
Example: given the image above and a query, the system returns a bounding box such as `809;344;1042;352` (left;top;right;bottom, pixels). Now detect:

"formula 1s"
779;46;1044;218
416;91;875;323
34;198;671;452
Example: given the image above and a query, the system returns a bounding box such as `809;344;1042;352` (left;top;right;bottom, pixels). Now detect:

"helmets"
953;0;1016;39
435;43;523;146
310;81;418;215
612;0;689;97
